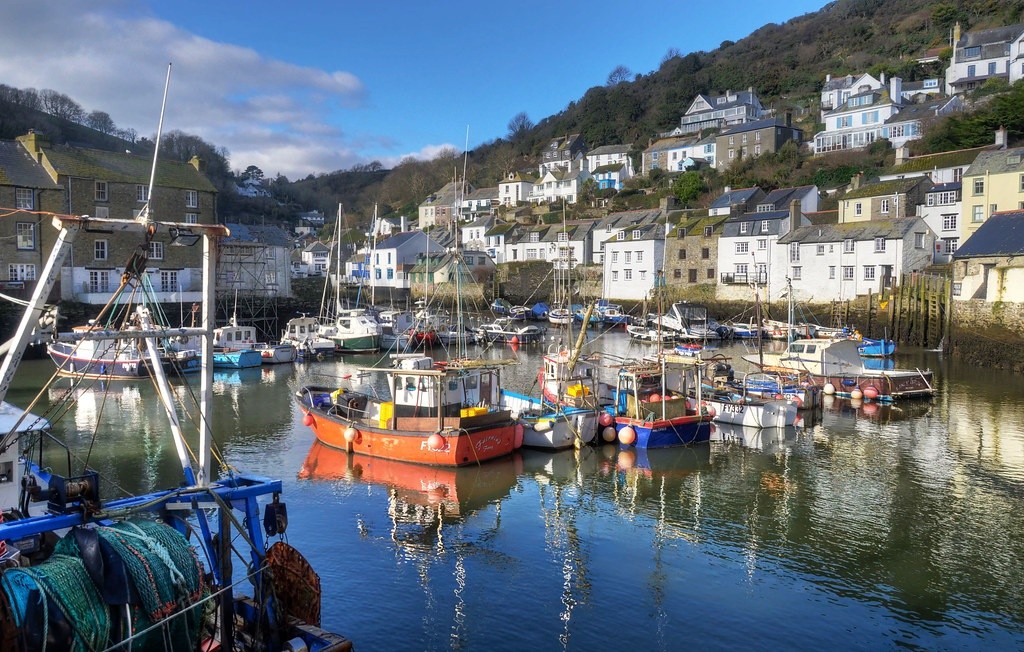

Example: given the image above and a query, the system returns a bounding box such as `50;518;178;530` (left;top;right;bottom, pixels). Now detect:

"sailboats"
588;270;717;449
603;443;709;629
315;202;383;354
295;246;524;466
296;439;524;649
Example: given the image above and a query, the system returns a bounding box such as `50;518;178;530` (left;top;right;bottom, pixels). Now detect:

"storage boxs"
566;384;589;397
603;387;629;416
460;407;488;418
379;401;394;429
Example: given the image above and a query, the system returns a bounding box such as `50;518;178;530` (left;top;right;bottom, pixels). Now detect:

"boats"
211;343;262;368
46;309;172;380
493;388;599;452
743;282;939;408
516;447;600;550
671;355;798;429
212;288;298;366
706;421;801;452
377;294;844;349
278;313;339;359
737;372;822;409
854;334;897;357
159;334;204;374
